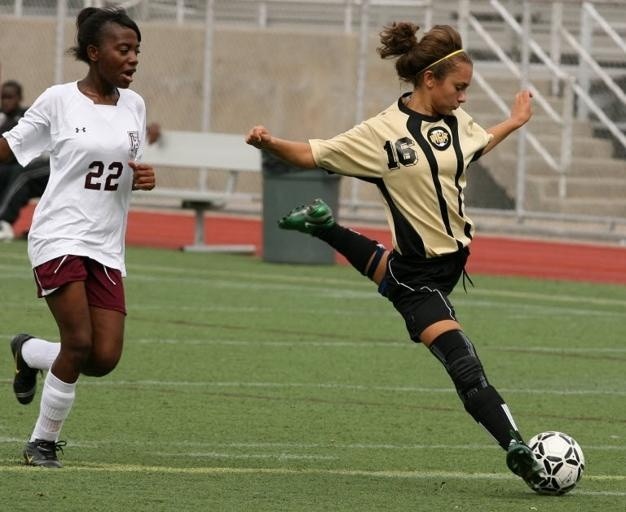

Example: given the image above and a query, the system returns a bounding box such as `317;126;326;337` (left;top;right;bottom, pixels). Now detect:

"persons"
0;6;156;467
244;21;549;488
0;80;49;240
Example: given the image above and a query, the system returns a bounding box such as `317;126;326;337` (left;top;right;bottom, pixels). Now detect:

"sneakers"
23;439;63;470
498;430;550;492
10;333;39;405
277;198;335;233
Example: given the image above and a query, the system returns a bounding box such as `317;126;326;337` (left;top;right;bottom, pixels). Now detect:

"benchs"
0;3;619;255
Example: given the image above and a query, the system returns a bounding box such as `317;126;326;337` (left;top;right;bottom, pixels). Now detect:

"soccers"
522;431;585;496
